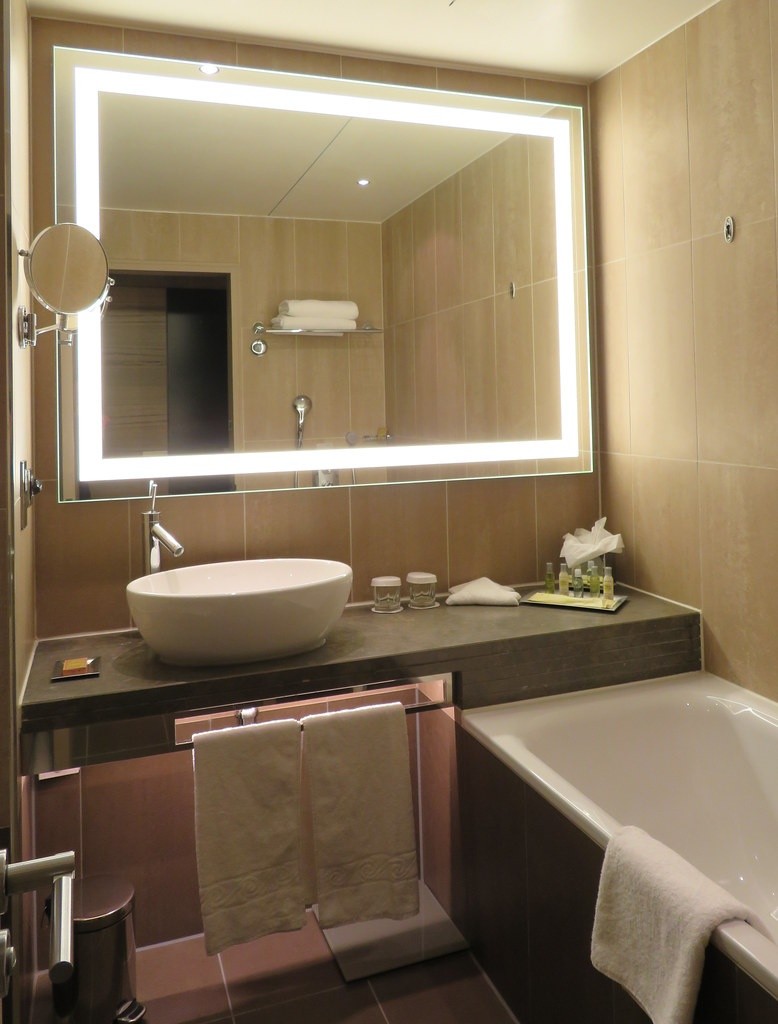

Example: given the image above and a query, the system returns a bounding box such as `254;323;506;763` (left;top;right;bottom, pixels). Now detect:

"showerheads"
291;394;313;451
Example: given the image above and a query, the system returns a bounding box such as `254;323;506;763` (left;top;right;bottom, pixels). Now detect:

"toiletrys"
545;562;556;594
573;568;583;599
586;559;597;582
588;565;600;598
602;567;615;600
558;563;569;597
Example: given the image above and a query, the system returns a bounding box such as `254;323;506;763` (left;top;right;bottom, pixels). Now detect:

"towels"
277;299;360;317
587;823;777;1024
299;699;422;933
445;574;523;607
189;715;311;959
270;315;359;336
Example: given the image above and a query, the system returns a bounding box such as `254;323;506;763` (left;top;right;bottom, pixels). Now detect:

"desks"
21;578;704;926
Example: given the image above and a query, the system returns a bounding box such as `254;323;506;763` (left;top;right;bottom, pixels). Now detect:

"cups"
407;574;436;607
374;576;401;612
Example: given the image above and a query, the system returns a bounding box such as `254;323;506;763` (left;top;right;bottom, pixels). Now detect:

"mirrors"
15;222;115;349
51;45;595;502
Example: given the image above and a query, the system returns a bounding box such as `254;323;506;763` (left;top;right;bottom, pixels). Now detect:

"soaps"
62;656;90;675
374;424;388;438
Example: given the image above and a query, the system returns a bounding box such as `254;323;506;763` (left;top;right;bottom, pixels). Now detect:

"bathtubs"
463;667;778;1024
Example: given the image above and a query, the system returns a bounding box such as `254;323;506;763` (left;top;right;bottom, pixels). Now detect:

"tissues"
559;517;626;570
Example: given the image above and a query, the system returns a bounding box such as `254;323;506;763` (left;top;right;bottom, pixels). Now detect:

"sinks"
125;556;354;670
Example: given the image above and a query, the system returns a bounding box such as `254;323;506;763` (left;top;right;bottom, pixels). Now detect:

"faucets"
139;483;185;580
